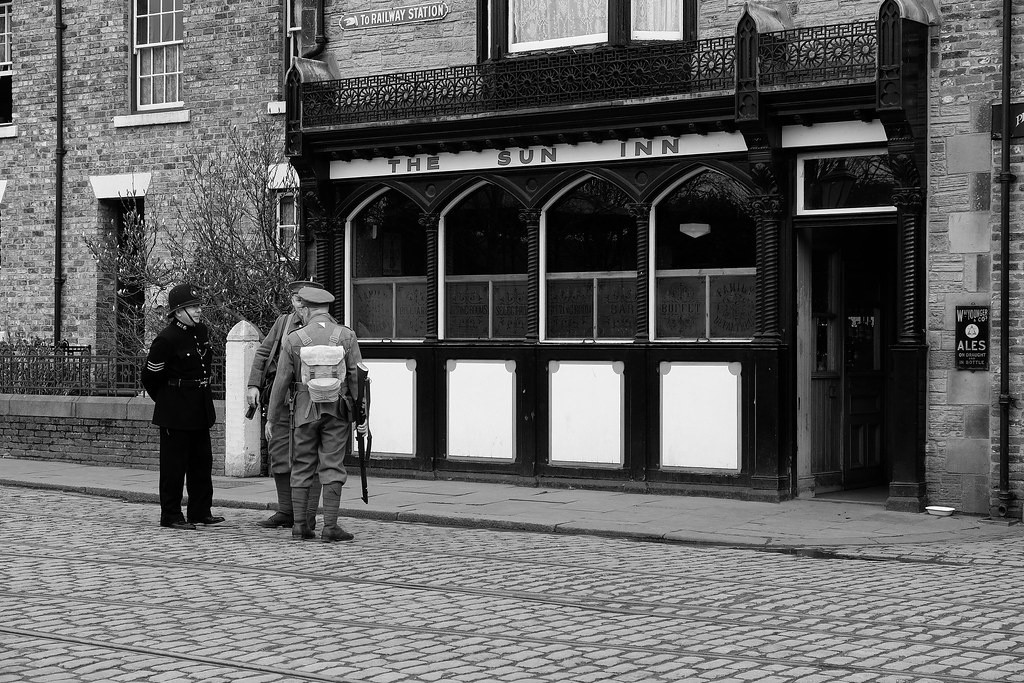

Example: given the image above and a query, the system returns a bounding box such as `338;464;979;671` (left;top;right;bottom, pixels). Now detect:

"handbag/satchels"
259;362;277;405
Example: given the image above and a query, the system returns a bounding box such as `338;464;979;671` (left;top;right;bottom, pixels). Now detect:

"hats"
297;287;335;308
288;281;325;298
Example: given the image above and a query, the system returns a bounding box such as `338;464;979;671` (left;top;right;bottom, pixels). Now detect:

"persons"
247;281;324;530
265;287;368;542
141;284;225;530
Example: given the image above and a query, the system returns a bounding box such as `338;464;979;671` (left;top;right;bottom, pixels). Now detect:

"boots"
256;473;294;528
320;482;354;543
291;487;315;541
306;473;322;531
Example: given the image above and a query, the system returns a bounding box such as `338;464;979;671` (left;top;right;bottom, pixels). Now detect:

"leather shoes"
187;516;225;524
171;521;196;530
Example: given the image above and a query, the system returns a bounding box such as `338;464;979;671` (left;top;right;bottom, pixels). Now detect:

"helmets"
167;284;206;326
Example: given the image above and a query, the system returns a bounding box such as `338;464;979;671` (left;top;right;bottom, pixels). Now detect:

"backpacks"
294;325;347;404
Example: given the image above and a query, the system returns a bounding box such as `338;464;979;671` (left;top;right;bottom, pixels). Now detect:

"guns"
245;388;262;420
354;361;371;504
